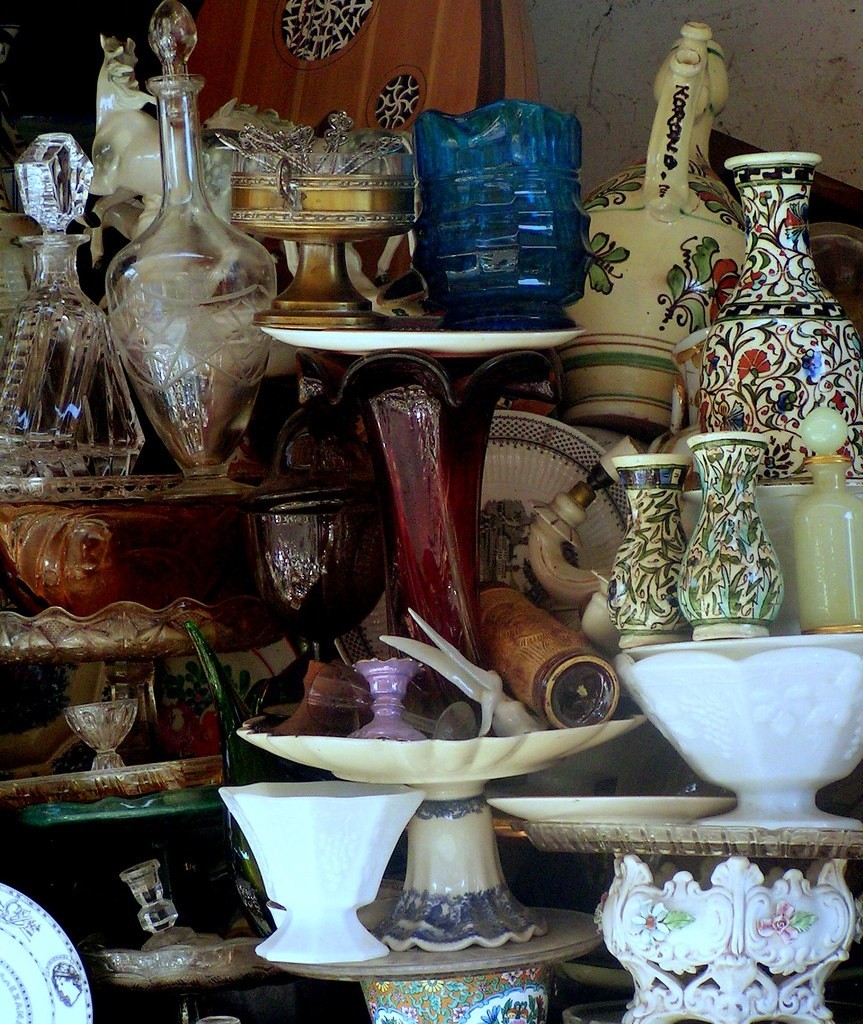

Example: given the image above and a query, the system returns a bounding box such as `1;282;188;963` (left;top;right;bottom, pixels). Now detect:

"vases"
598;142;862;645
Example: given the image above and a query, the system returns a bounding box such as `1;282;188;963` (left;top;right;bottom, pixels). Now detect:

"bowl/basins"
614;646;862;830
219;780;425;964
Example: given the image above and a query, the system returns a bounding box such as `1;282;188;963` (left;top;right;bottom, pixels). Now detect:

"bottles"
612;454;693;648
0;132;145;475
793;407;863;635
698;151;862;480
687;432;784;641
103;3;275;500
184;621;300;938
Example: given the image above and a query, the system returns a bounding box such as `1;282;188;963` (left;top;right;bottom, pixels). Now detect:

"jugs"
562;22;747;432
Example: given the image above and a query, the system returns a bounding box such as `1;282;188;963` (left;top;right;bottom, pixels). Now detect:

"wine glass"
63;699;138;771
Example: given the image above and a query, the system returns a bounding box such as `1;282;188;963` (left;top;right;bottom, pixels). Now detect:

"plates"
0;883;93;1024
486;796;736;828
333;409;629;665
262;325;585;357
621;633;863;660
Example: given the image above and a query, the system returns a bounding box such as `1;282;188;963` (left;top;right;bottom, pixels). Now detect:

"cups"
475;580;620;729
196;1016;241;1024
